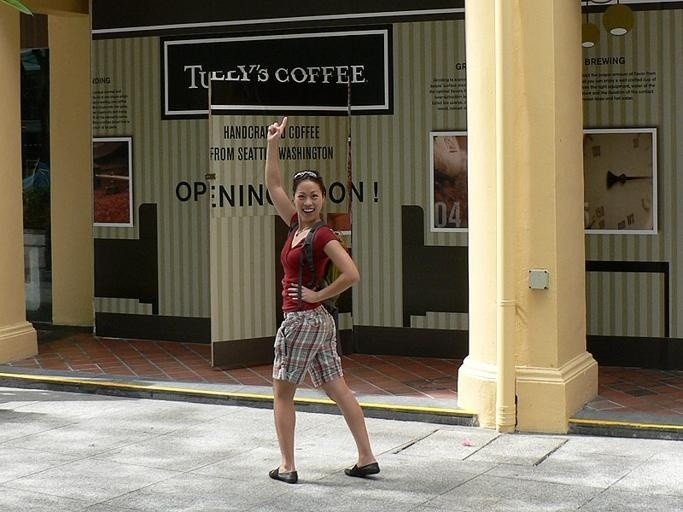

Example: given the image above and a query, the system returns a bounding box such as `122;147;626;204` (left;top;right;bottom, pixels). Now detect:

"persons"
266;116;380;484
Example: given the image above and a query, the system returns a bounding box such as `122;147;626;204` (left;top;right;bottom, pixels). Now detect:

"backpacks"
287;222;348;312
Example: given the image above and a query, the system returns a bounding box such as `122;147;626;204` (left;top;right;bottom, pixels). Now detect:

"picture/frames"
427;124;661;236
89;135;136;228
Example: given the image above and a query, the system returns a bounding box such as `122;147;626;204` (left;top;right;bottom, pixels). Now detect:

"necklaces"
296;228;309;237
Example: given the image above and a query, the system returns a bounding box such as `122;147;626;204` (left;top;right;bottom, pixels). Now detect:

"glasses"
294;171;318;180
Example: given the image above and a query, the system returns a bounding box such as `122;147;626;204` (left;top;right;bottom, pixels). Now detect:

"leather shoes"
344;462;380;477
268;466;298;483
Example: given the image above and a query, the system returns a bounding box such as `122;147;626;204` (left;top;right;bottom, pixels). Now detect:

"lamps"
581;0;634;48
